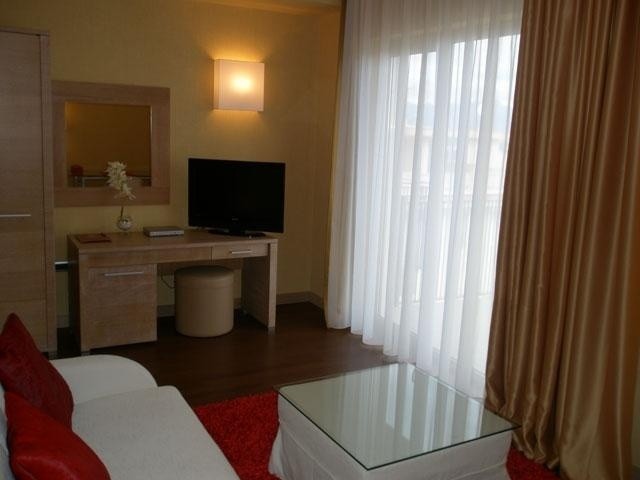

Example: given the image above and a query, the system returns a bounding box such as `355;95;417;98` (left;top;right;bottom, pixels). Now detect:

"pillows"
2;315;111;478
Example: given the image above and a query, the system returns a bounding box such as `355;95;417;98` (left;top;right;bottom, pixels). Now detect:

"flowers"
106;162;136;218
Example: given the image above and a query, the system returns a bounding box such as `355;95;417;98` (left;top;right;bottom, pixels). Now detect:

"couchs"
0;353;243;479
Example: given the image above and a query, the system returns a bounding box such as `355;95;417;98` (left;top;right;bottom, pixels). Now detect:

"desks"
67;228;278;351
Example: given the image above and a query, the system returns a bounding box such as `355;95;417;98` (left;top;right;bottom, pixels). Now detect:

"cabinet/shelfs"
0;27;58;359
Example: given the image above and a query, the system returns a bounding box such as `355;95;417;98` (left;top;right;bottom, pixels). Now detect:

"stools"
175;266;234;337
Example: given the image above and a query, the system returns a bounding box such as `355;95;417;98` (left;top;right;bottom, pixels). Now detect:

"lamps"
214;59;264;111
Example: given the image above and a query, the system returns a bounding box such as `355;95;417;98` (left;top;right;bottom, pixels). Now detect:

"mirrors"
52;80;171;206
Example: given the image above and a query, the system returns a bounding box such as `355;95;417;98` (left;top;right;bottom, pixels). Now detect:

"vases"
118;217;133;237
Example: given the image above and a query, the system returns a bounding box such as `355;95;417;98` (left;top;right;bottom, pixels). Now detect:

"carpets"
200;390;534;478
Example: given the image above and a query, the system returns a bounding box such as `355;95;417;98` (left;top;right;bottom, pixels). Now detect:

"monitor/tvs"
188;158;288;238
